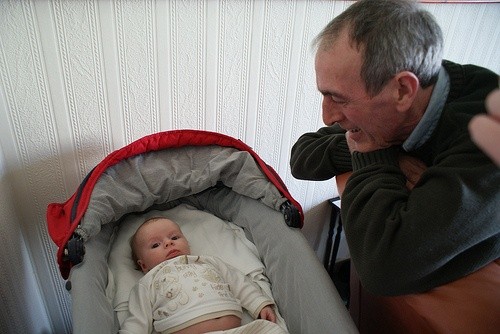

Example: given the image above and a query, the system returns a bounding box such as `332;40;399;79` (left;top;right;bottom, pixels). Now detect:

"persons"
290;0;499;298
469;89;499;165
117;217;288;334
